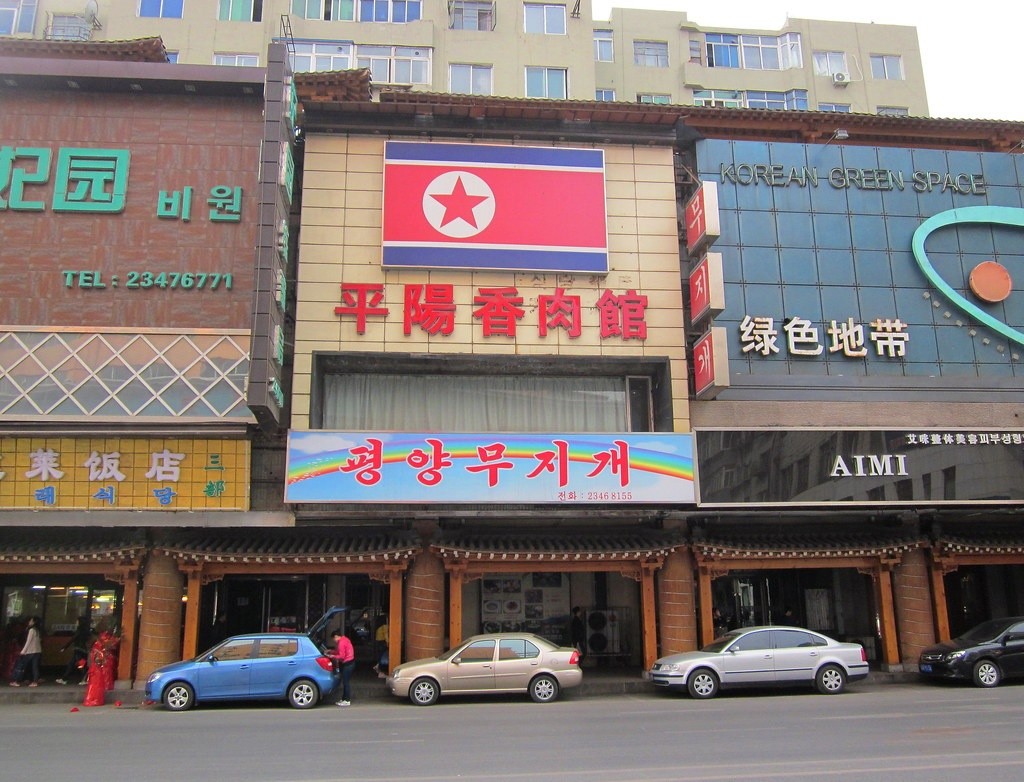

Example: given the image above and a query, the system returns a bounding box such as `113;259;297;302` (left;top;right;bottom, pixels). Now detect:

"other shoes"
10;681;20;687
378;672;387;679
28;682;38;687
56;678;67;685
78;680;88;686
373;664;379;673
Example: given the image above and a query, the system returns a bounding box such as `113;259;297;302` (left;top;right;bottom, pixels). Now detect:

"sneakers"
335;699;350;706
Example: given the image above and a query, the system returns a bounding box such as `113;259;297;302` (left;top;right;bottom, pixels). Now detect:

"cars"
918;617;1024;688
144;606;351;711
387;632;583;707
649;626;869;700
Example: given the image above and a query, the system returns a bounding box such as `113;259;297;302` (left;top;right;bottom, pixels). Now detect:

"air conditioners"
835;70;853;88
582;609;622;654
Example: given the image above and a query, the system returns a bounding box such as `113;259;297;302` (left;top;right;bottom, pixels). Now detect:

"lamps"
1008;139;1024;154
825;127;849;145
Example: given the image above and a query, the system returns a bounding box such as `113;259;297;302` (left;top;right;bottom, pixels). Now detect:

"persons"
373;619;389;678
572;607;587;669
56;616;90;685
324;631;355;707
9;616;42;687
713;608;721;639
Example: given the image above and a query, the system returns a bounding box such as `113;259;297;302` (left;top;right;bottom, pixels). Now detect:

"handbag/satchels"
571;642;583;656
